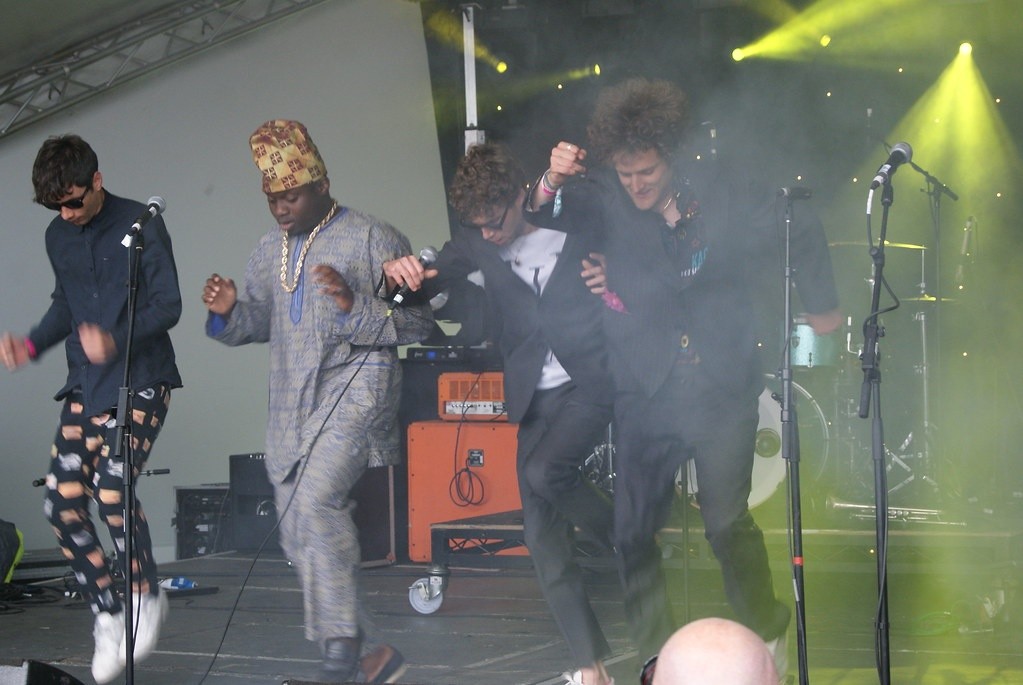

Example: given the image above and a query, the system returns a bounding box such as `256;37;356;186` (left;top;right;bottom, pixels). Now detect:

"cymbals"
828;239;930;250
899;293;954;303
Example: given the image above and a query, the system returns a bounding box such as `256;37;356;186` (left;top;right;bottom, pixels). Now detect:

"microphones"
387;246;438;316
127;195;167;237
957;221;972;276
870;141;912;190
779;186;812;200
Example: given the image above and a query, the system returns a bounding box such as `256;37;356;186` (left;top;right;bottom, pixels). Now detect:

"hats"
250;120;328;193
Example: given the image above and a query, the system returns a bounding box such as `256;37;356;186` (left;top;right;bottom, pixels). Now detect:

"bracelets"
541;169;561;195
23;336;36;359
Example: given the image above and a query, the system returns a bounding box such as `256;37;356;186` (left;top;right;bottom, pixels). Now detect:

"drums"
670;374;829;524
784;311;852;374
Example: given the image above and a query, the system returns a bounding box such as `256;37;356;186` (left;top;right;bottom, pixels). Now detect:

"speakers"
0;657;85;685
228;452;283;551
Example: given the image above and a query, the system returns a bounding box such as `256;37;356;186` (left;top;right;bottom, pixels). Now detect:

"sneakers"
120;585;168;667
91;600;126;685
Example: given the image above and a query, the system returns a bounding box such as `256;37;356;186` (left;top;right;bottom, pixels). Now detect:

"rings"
567;143;571;150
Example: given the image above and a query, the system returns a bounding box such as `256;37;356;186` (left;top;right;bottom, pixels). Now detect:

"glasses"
460;198;509;230
42;173;93;210
640;655;659;685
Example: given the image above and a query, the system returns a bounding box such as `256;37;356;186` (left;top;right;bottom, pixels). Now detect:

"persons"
375;143;616;685
650;616;779;685
0;135;184;685
521;77;841;685
200;120;434;685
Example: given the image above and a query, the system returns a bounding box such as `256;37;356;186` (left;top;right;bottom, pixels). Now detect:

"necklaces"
663;196;673;210
280;199;337;293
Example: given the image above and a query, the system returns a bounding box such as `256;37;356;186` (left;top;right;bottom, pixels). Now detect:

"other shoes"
768;632;789;680
561;671;614;685
322;626;362;682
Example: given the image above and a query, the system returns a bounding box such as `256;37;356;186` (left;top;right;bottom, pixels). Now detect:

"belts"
675;351;700;365
